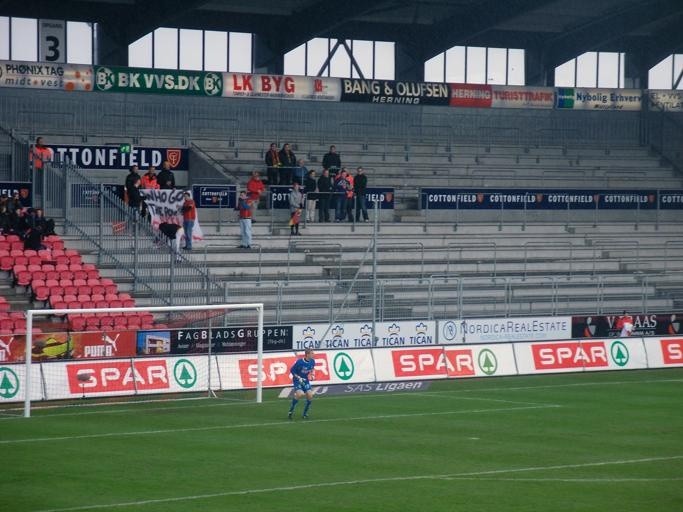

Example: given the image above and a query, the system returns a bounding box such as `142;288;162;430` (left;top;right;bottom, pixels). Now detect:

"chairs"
0;210;171;335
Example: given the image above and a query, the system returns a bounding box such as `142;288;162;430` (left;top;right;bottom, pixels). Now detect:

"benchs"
46;134;680;326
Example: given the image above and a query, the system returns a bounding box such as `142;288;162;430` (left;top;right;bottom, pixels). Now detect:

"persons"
159;223;184;253
288;349;315;417
126;165;142;188
0;186;54;251
29;137;51;194
141;166;160;189
181;193;196;249
127;178;147;229
237;191;253;249
157;161;175;189
617;310;633;337
265;143;369;236
246;170;266;222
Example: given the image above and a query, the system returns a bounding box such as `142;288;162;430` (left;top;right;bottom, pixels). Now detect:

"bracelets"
297;376;300;381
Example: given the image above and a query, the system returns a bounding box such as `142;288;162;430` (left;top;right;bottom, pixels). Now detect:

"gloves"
297;376;306;386
311;374;314;381
